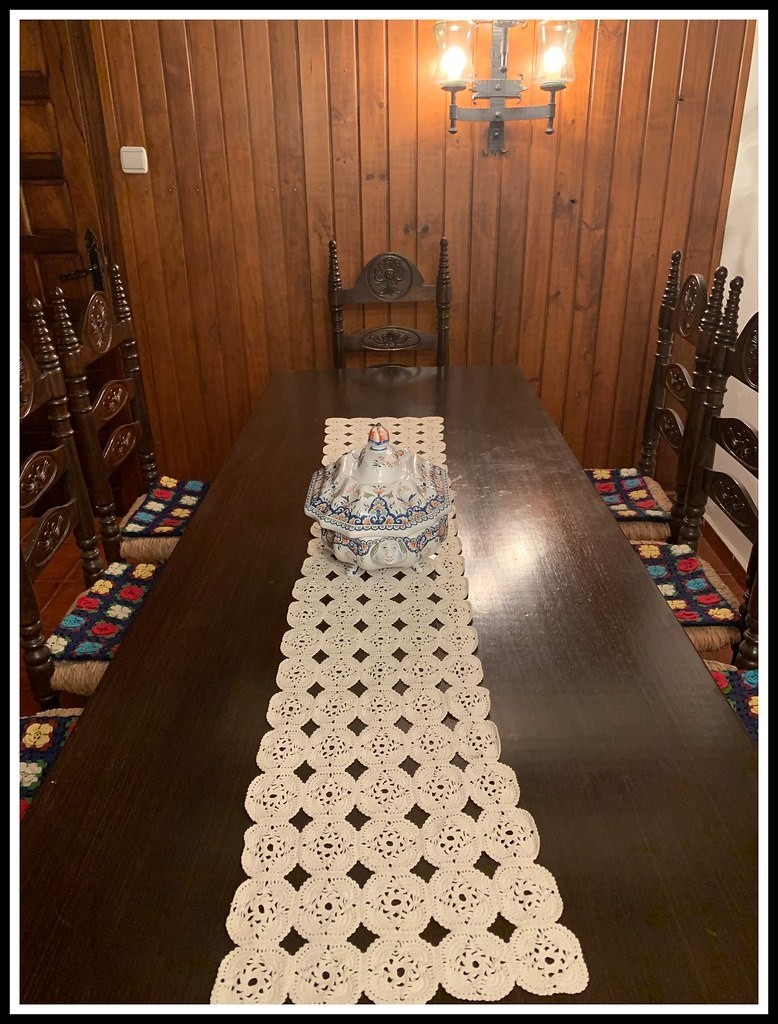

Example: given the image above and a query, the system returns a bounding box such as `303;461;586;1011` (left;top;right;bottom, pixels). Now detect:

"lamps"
433;20;578;155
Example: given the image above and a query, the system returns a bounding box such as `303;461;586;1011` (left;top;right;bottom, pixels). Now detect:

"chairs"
51;262;210;564
583;251;727;539
704;575;758;748
19;297;166;711
19;708;83;821
328;237;451;369
628;276;758;666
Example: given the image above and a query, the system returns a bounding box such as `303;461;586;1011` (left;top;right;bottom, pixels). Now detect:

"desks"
19;368;757;1005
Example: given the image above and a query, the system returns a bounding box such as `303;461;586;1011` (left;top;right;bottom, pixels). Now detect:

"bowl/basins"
306;424;453;571
